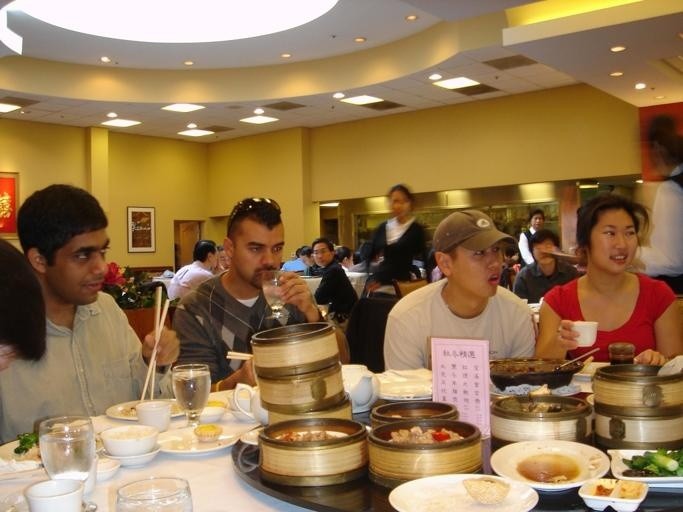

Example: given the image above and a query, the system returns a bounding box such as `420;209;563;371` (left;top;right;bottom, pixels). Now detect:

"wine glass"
259;268;288;321
171;361;210;424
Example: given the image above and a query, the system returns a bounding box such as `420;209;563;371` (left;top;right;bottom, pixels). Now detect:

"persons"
213;247;231;275
627;117;682;296
0;237;46;374
498;211;580;305
279;238;384;364
384;212;535;372
361;184;428;373
170;198;350;392
1;184;180;446
167;240;218;300
535;193;682;372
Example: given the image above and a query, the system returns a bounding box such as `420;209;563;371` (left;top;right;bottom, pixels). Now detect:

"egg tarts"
194;424;223;442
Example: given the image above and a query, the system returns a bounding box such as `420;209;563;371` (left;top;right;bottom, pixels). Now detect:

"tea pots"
338;362;379;416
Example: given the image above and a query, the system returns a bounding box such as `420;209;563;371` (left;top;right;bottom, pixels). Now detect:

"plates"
386;470;541;512
576;477;650;512
488;437;608;494
604;445;682;490
572;361;610;380
370;381;432;400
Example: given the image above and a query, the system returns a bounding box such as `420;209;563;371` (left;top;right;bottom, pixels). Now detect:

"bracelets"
216;380;222;391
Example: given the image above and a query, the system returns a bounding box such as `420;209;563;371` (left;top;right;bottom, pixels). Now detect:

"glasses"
225;198;281;230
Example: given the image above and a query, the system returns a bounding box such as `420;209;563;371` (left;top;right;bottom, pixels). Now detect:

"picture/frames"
0;167;24;245
348;197;563;253
125;205;157;254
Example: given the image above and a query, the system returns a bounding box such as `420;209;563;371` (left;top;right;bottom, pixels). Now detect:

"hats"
432;209;519;252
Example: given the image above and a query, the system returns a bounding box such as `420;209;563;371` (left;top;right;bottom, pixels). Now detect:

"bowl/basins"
485;358;586;390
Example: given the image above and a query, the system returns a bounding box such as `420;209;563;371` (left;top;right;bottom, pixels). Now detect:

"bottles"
606;340;635;365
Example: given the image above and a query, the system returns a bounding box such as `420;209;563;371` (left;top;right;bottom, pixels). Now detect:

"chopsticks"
138;284;170;403
582;356;594;373
224;351;252;361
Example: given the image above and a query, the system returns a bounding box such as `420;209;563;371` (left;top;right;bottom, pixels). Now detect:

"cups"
22;382;268;511
568;319;598;348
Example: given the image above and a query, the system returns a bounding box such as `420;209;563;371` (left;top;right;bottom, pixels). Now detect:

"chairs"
0;248;683;512
